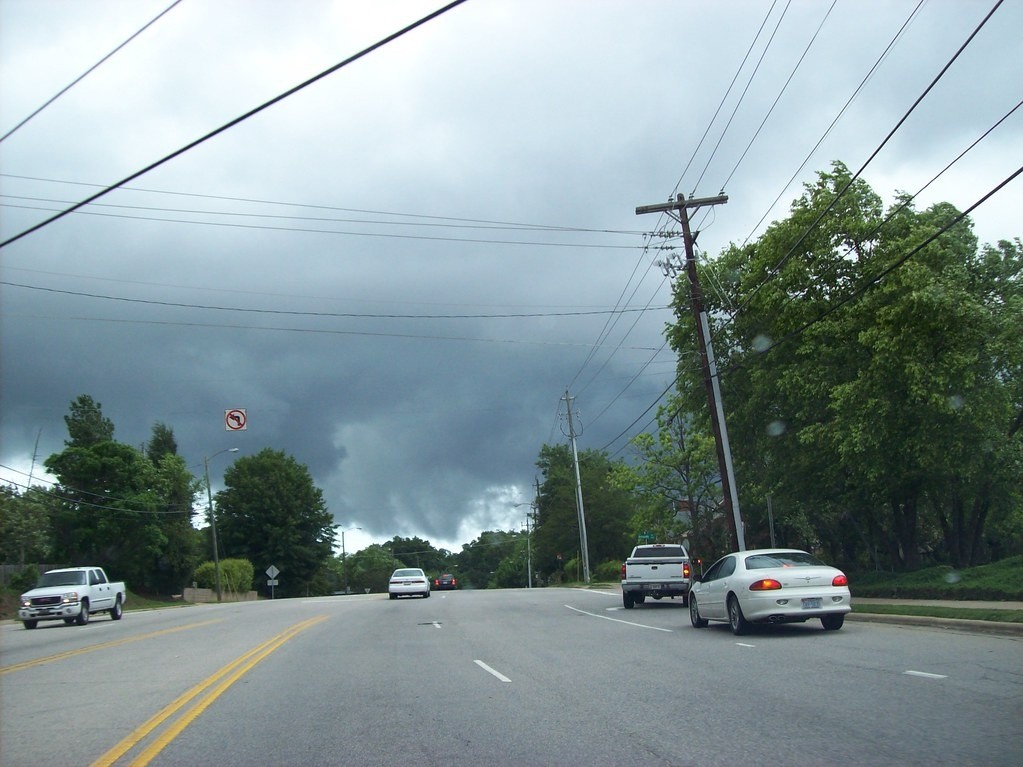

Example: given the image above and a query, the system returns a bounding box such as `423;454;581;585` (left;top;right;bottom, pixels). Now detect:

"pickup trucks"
18;566;127;629
621;543;700;610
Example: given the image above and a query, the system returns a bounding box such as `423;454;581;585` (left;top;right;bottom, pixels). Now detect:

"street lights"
514;502;537;532
204;447;239;603
342;527;363;595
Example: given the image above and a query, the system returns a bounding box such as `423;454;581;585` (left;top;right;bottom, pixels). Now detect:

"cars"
388;567;431;599
435;574;461;590
687;548;852;636
